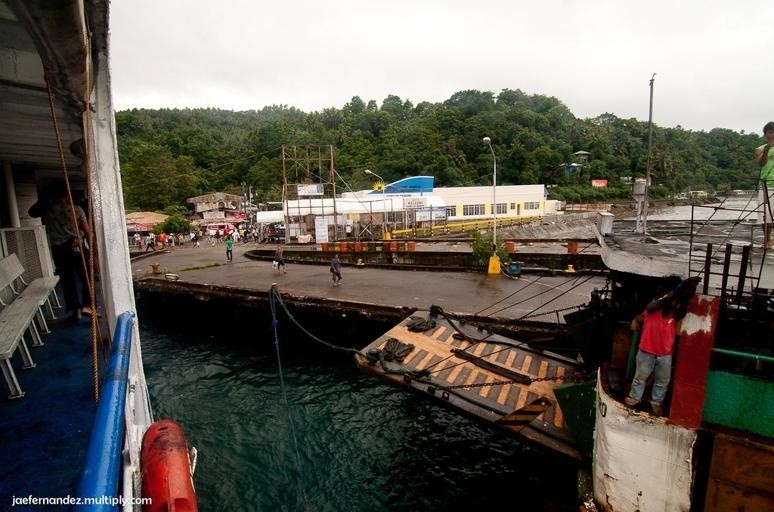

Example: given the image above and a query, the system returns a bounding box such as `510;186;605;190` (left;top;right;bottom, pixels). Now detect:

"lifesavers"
140;419;198;512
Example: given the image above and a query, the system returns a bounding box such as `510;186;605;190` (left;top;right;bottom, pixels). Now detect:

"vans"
205;223;237;238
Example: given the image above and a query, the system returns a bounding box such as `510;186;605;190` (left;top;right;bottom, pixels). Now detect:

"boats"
354;204;774;512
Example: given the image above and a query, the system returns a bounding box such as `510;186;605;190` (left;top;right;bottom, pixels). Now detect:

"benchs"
0;253;60;400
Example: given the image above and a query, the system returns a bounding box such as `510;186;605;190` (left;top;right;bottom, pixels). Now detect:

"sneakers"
333;282;341;287
624;395;663;416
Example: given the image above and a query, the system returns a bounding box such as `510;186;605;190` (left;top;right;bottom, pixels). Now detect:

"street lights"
365;170;388;232
642;73;658;235
482;137;497;256
249;184;254;207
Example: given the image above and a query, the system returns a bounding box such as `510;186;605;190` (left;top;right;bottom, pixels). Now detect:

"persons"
274;246;287;274
624;282;689;416
754;122;774;248
208;224;260;247
28;176;101;323
193;233;200;249
133;231;174;253
331;254;343;287
357;258;365;265
226;237;233;262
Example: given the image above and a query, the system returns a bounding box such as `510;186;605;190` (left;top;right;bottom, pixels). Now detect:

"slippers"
81;311;102;319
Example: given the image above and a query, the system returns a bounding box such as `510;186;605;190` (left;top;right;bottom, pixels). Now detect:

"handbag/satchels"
330;265;336;272
